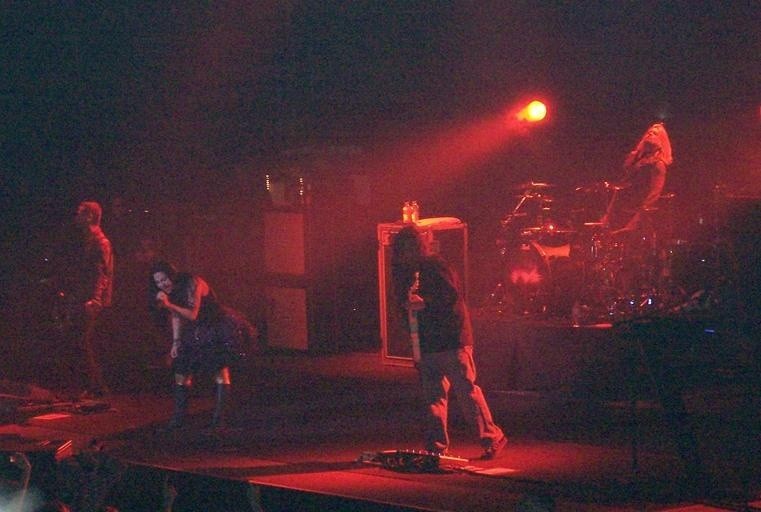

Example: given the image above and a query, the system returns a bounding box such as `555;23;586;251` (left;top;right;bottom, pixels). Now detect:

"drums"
499;240;586;322
593;241;633;269
640;239;685;266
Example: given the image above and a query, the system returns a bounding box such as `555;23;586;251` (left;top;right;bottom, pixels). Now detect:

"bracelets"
172;338;181;346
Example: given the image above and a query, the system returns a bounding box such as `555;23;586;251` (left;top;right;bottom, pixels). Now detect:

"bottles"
403;201;419;226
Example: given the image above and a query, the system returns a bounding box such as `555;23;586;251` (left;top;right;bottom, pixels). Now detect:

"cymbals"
512;192;558;204
509;181;558;190
519;227;579;245
575;181;632;195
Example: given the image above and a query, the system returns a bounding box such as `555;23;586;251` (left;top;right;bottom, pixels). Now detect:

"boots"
168;383;190;428
211;382;232;429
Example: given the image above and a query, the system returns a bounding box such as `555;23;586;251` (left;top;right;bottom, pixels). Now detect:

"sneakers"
480;435;509;460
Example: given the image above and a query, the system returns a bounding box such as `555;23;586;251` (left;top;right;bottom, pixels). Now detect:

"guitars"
408;273;422;374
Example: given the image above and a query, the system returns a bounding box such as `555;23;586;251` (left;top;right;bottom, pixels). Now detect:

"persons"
391;225;509;459
50;202;114;407
597;123;673;239
147;258;257;432
